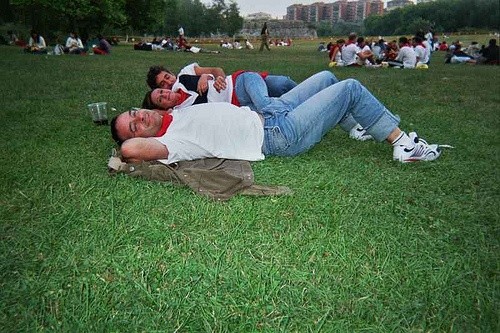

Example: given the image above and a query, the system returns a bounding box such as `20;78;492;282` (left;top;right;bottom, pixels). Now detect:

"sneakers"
349;123;373;141
392;131;443;163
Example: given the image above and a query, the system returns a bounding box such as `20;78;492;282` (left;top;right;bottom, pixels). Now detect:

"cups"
87;101;109;125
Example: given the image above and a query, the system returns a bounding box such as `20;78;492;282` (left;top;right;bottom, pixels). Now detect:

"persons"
110;70;442;166
9;27;129;56
316;26;500;72
132;19;293;54
141;62;298;115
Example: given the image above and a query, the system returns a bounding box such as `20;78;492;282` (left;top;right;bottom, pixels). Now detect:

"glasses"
131;107;137;137
